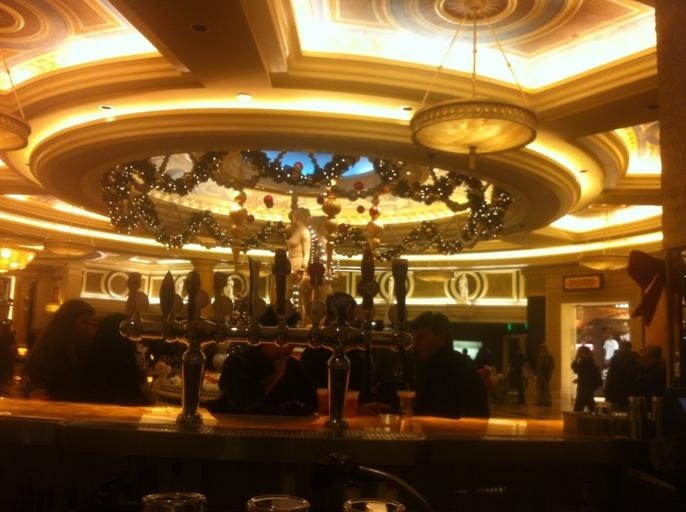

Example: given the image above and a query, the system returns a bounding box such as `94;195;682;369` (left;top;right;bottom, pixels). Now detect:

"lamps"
409;0;539;154
578;203;630;270
0;244;40;273
44;203;96;256
0;38;31;152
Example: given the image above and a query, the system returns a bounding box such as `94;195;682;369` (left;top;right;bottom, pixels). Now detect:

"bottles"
176;339;207;427
325;335;352;430
628;395;662;440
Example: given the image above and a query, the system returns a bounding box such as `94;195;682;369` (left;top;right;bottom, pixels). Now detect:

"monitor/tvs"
450;338;484;360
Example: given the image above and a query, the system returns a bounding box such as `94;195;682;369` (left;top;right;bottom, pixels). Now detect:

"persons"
20;297;95;405
476;340;556;403
301;290;374;396
411;310;487;417
213;299;318;414
571;332;668;411
64;310;165;406
285;207;311;271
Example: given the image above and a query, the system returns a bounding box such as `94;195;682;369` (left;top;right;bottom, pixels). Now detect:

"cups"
317;388;330;415
344;391;359;417
397;390;415;417
